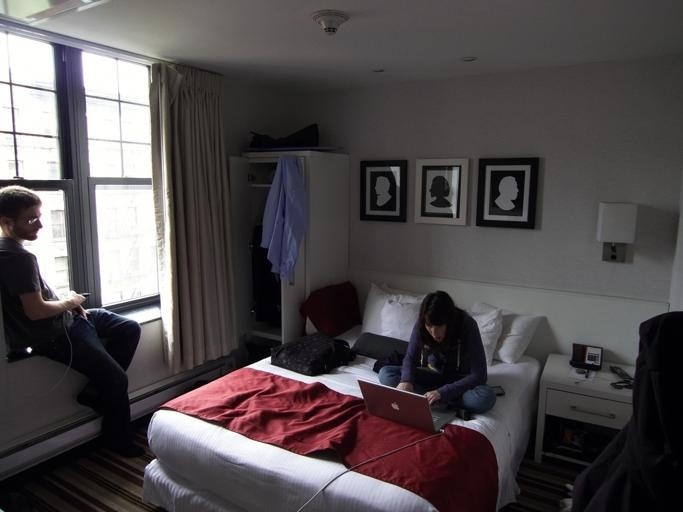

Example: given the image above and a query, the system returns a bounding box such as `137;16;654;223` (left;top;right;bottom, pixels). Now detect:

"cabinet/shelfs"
227;151;350;348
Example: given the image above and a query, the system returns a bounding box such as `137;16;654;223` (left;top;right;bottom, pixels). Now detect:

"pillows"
351;332;409;361
360;278;546;371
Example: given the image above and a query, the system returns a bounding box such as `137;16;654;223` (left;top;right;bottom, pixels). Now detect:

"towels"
259;153;311;286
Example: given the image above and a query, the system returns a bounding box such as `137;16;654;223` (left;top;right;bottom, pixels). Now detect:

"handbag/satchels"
270;334;356;376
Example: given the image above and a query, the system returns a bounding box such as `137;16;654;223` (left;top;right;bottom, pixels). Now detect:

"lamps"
594;200;640;264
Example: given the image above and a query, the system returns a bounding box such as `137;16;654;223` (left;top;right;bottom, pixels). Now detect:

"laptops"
357;378;456;434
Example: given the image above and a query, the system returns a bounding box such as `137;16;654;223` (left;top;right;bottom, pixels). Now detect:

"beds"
143;354;540;512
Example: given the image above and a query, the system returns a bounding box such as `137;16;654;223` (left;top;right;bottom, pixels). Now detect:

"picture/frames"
413;158;470;227
475;155;540;230
358;158;409;222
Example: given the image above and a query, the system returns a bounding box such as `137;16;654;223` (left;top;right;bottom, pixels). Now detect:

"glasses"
610;379;633;390
28;216;38;225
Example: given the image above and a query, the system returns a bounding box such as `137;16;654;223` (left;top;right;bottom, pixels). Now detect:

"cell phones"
78;292;93;297
491;386;505;396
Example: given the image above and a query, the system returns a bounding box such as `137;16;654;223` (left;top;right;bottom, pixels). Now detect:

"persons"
0;185;141;446
376;291;496;420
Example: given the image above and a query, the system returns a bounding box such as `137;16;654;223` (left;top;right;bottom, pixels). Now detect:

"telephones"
569;343;603;371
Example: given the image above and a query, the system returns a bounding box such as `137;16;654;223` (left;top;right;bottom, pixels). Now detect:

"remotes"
611;366;632;380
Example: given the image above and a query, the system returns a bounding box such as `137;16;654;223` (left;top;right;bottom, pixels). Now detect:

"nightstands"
533;351;638;463
